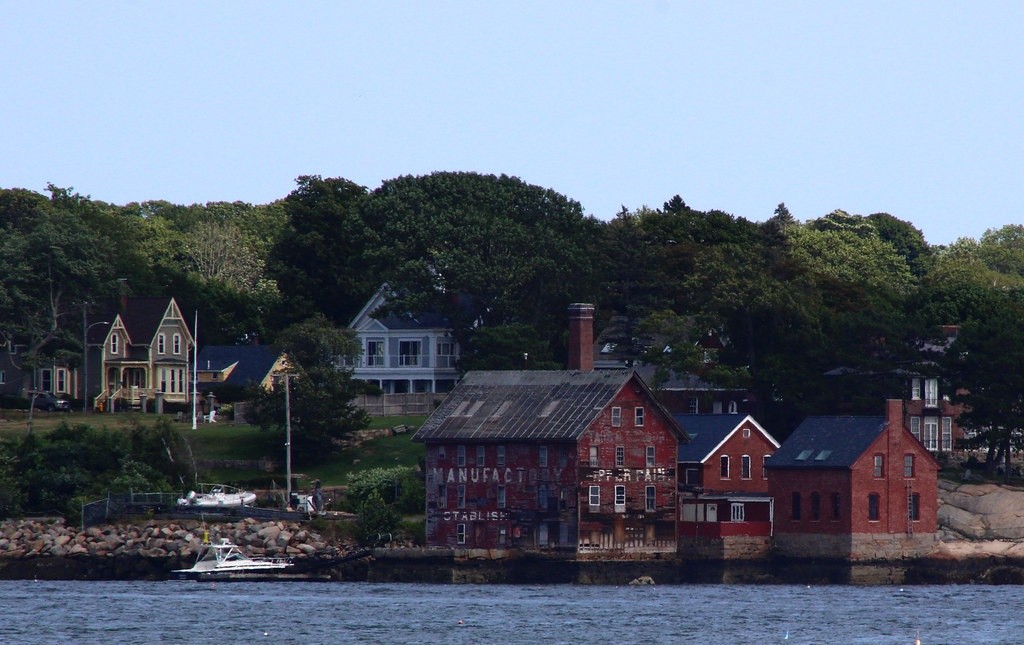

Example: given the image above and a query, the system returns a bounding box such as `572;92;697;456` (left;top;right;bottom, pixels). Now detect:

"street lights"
82;321;110;412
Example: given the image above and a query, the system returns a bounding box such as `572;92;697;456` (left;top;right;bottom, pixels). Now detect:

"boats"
169;538;295;584
180;482;256;510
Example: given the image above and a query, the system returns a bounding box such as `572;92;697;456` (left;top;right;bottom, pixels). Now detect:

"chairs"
203;411;216;424
197;411;203;422
173;412;183;422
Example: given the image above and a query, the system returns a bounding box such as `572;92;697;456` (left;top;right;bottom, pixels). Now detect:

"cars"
27;391;71;413
